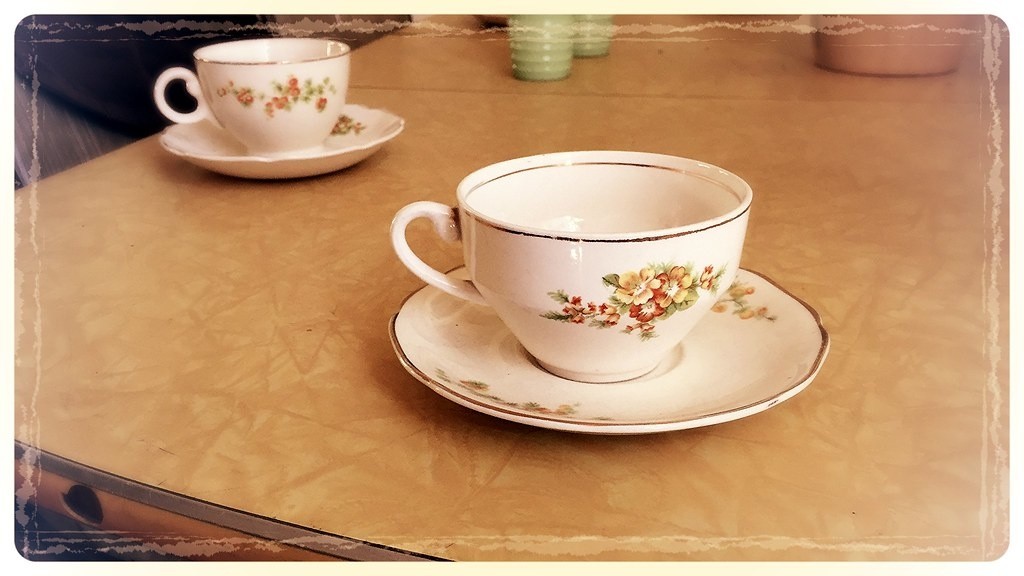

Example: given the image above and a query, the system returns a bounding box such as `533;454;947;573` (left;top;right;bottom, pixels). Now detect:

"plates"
158;104;406;179
390;265;831;435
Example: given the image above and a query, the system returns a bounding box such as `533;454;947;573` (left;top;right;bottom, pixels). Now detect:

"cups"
813;15;977;76
153;38;350;154
509;15;572;81
390;151;754;377
572;15;612;56
